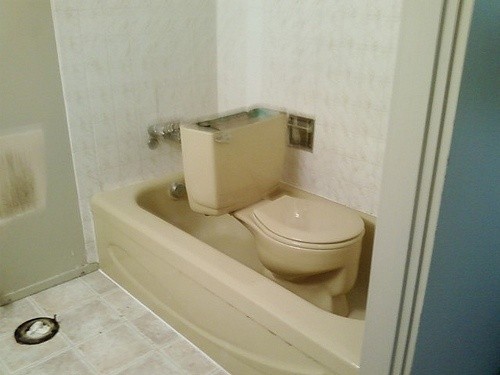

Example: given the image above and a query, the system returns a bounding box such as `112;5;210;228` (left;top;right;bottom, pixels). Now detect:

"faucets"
148;119;182;148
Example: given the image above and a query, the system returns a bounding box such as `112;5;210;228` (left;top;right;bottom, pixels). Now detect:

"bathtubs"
90;173;377;375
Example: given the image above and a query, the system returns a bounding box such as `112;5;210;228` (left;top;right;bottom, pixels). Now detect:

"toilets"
179;104;367;317
